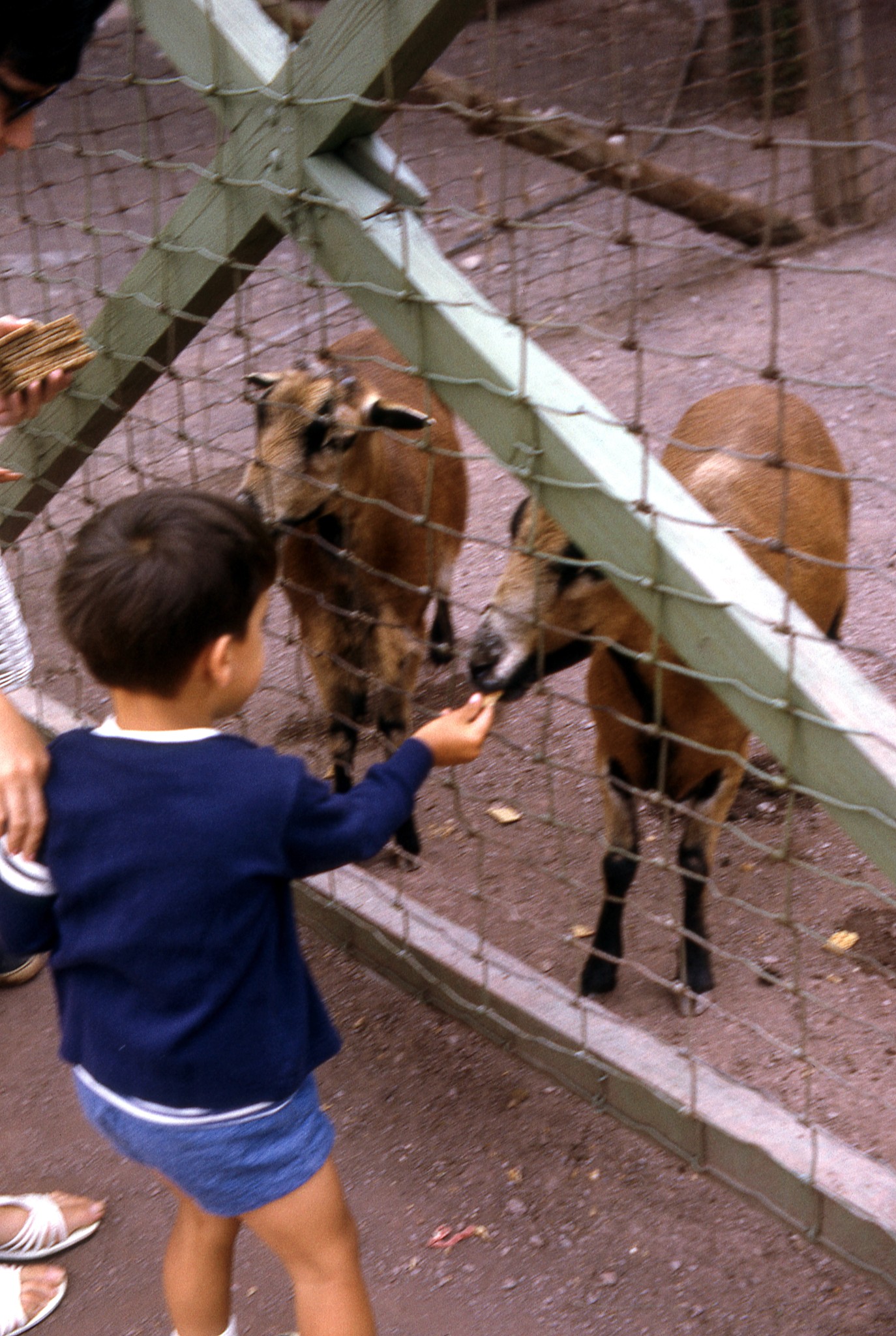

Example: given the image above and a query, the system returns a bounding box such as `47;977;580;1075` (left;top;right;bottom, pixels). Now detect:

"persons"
3;1;138;991
0;484;515;1336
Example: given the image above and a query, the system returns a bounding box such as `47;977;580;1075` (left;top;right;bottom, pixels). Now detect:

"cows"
229;326;471;867
467;386;846;1019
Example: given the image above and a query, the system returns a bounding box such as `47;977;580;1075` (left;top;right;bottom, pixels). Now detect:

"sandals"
0;1190;100;1260
1;1262;70;1336
